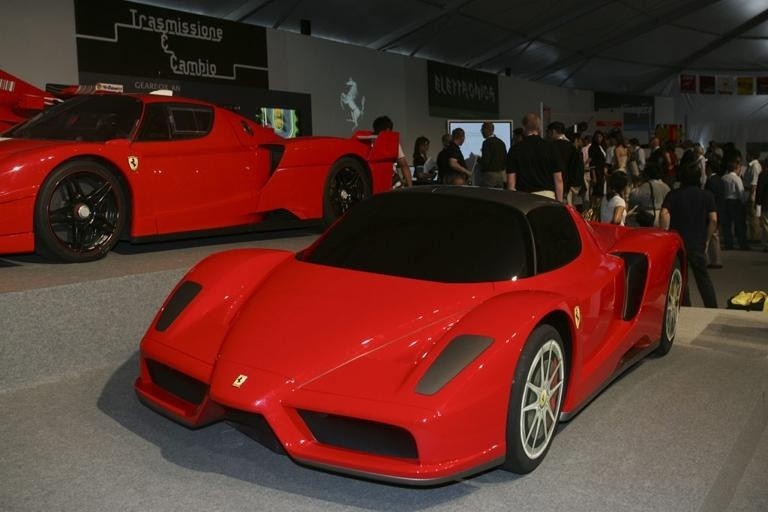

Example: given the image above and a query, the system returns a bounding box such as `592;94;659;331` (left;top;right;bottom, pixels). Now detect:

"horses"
339;77;366;131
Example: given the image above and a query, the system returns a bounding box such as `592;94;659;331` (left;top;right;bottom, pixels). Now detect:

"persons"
372;114;592;215
588;130;767;309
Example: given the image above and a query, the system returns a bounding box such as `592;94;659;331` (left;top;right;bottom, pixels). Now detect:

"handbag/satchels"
637;211;655;227
568;148;585;186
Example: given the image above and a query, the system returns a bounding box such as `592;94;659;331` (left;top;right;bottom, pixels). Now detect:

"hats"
603;128;620;136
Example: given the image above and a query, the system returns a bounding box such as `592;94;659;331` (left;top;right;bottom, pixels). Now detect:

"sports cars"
0;92;398;265
135;185;686;483
